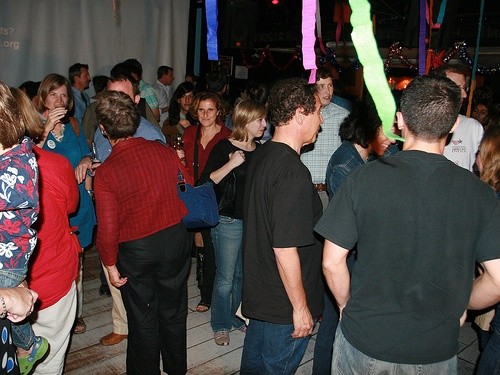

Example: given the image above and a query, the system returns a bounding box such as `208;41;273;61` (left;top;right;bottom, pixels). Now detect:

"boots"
196;246;202;289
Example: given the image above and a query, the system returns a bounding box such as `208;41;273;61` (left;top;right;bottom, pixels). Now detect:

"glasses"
458;86;469;92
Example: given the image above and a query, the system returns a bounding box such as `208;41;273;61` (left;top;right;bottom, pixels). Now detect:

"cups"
54;100;70;124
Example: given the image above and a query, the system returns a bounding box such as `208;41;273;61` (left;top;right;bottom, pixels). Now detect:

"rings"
26;311;31;316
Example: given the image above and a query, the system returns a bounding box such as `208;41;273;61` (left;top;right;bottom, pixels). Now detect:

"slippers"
197;301;211;312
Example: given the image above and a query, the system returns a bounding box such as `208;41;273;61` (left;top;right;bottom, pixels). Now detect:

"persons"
0;58;500;375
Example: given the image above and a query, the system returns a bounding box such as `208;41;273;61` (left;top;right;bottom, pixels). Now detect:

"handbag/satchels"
175;169;218;228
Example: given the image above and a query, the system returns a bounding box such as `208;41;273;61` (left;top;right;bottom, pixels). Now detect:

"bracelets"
0;294;6;318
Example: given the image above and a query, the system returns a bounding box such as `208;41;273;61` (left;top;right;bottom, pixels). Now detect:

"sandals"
16;336;48;375
72;317;85;333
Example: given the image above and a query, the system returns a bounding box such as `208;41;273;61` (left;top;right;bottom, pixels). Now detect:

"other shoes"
99;332;129;346
99;284;110;298
213;330;229;346
237;321;249;332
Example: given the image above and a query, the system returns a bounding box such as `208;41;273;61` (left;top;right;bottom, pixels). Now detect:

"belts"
315;183;326;191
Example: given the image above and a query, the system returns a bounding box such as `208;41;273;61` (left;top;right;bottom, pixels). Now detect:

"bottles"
91;143;101;165
175;133;183;151
